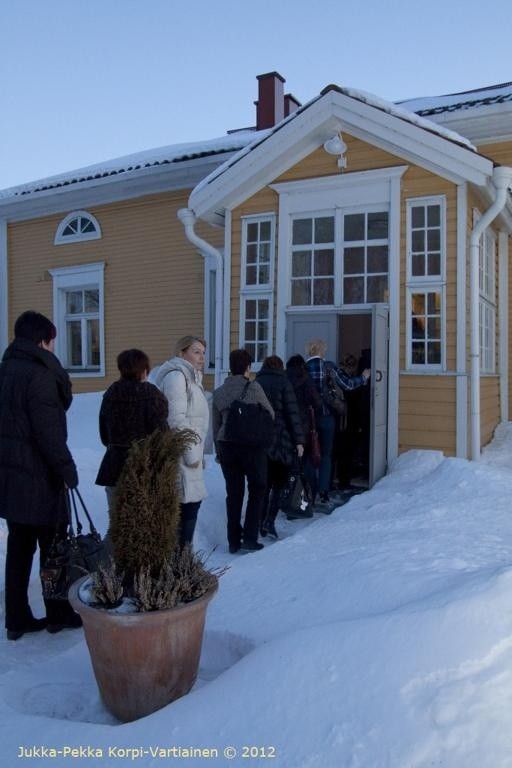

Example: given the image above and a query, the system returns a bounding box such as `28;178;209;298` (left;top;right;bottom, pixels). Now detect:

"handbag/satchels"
224;399;274;446
287;473;314;518
322;372;349;414
40;531;111;599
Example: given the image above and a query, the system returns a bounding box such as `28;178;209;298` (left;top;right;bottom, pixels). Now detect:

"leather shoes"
229;540;264;553
319;490;330;503
260;522;278;539
8;611;83;640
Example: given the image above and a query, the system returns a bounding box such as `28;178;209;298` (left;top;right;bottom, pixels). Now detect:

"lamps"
323;128;348;169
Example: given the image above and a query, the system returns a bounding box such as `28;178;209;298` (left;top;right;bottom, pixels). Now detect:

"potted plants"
67;428;232;723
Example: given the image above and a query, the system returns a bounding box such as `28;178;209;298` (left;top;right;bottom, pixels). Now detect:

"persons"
95;347;171;526
211;348;275;554
302;336;372;505
147;335;209;556
327;359;356;492
0;309;83;640
335;352;359;490
251;355;306;539
280;354;320;520
